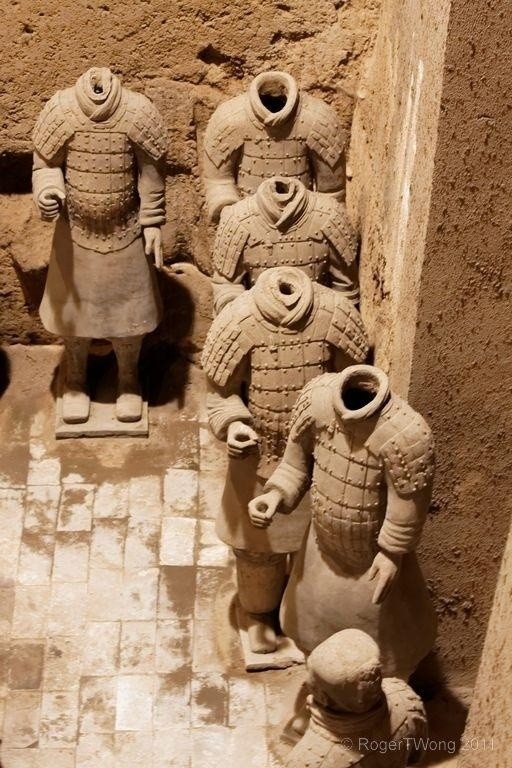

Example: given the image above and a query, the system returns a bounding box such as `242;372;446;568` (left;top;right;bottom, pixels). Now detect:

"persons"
197;265;371;654
208;176;362;315
27;65;169;421
244;362;439;689
285;626;433;767
201;70;351;226
